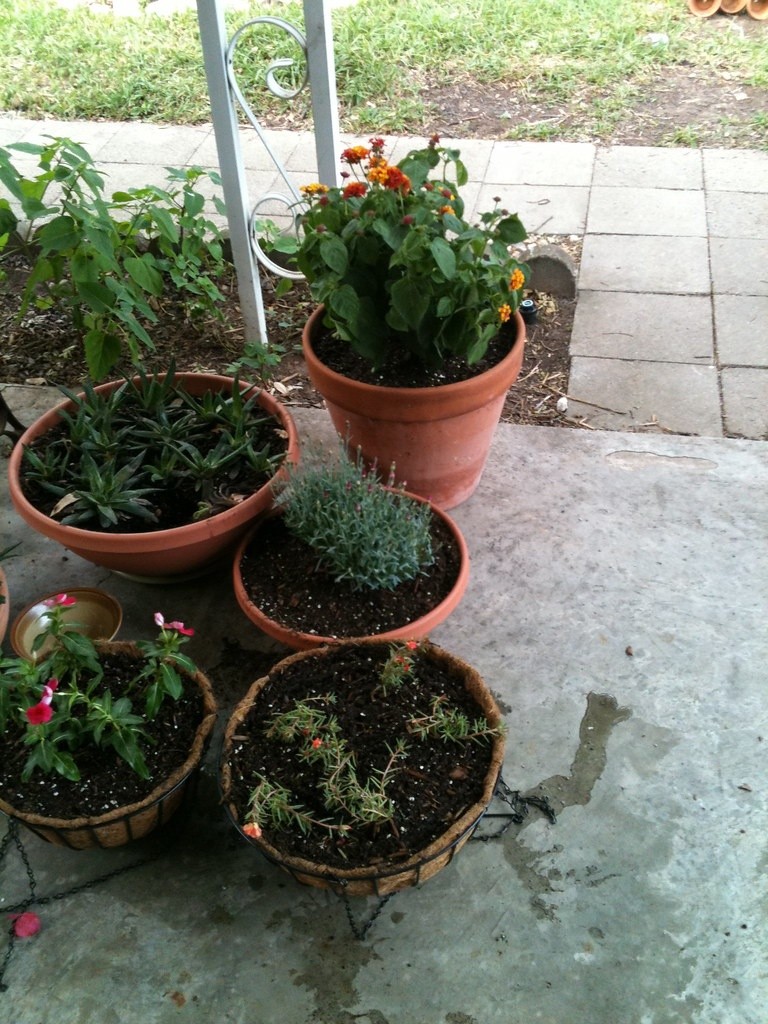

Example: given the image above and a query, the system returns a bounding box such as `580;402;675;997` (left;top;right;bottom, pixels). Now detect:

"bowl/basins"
8;587;123;665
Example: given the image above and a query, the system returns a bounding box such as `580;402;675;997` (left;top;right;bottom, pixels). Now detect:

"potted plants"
0;133;299;584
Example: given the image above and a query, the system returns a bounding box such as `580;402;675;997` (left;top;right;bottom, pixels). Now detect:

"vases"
218;639;508;899
234;485;471;651
301;304;526;511
0;640;216;852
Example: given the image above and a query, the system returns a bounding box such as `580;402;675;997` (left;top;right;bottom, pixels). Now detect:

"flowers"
287;129;534;365
263;417;432;595
0;594;195;783
237;640;511;840
